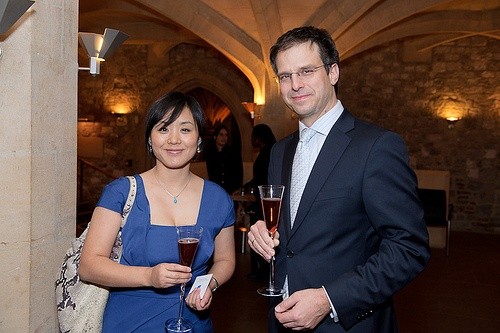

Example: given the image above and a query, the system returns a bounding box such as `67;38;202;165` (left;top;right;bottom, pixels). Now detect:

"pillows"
418;188;446;226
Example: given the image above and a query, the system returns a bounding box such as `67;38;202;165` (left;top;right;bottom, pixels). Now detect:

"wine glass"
257;185;285;296
165;227;204;333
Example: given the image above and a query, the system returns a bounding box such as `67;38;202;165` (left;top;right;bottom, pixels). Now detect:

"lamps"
241;102;256;118
78;28;129;77
446;118;459;128
112;112;124;119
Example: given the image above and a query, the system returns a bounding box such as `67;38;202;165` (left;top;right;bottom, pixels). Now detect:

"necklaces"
153;166;191;203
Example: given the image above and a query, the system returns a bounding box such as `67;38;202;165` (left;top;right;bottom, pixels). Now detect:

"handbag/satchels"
54;176;138;333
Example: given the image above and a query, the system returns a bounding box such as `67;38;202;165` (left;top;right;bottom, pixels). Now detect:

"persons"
77;92;236;333
207;124;244;195
248;25;430;333
241;124;278;280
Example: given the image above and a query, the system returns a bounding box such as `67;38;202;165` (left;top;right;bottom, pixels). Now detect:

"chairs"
413;170;450;257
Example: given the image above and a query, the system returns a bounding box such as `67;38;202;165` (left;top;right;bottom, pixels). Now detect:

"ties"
290;128;318;229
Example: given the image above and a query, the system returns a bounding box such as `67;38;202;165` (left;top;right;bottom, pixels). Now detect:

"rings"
251;238;256;245
191;304;197;311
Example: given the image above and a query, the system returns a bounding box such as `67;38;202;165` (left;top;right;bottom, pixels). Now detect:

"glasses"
273;62;334;84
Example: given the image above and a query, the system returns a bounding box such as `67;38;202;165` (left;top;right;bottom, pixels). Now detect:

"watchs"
211;276;220;293
